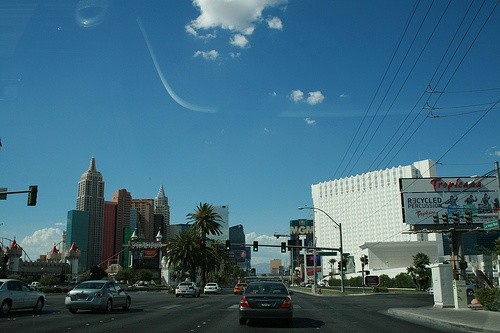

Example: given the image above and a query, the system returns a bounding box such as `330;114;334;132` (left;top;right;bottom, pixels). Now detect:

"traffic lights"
281;242;286;253
226;240;230;250
253;241;258;252
364;254;368;265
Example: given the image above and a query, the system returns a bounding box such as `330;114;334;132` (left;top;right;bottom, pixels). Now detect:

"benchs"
251;289;282;294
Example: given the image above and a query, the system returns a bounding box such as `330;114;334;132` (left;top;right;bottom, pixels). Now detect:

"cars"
233;283;247;295
239;281;294;322
175;282;200;298
64;280;132;312
204;283;222;294
0;279;48;316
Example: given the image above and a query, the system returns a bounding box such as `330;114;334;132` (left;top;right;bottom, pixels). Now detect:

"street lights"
298;206;344;293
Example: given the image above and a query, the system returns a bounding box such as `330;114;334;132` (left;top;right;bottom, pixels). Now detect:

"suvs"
427;282;474;296
